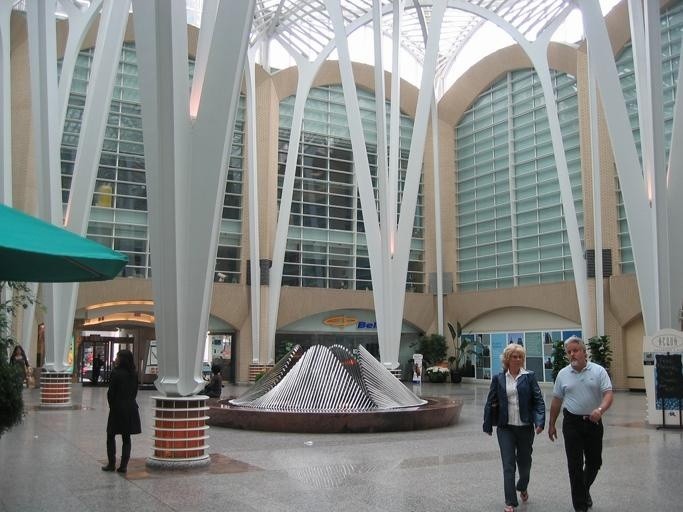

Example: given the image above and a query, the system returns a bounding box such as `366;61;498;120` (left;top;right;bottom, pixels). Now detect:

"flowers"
424;365;449;380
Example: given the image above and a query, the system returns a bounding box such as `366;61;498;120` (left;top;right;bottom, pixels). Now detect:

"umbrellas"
0;202;130;289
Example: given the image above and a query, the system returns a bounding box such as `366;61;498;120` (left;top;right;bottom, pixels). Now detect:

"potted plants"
447;319;487;384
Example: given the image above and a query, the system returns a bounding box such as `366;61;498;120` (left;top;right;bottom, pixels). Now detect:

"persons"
92;354;105;381
101;350;142;472
482;343;544;512
10;346;29;370
548;337;612;512
202;365;222;398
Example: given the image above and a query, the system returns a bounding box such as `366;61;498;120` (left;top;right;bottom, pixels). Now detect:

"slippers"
504;505;513;512
520;491;528;501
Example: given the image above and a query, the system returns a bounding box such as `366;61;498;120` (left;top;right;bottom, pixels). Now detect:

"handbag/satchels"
490;398;499;426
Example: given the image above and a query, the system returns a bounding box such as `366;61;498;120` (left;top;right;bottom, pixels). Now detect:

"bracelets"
597;407;604;417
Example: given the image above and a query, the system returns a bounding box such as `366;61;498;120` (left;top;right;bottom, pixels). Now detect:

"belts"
580;416;590;420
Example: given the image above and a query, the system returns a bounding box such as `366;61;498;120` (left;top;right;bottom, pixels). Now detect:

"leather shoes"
586;493;592;507
101;465;115;471
117;468;126;473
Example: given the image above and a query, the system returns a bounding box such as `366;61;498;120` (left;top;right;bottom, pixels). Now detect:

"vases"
429;374;445;382
